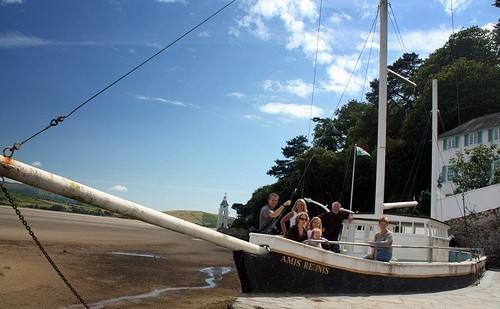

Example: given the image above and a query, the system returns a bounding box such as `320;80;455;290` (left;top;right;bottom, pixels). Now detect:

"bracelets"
282;204;286;208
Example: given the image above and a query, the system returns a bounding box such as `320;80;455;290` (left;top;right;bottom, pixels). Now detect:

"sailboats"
0;0;489;294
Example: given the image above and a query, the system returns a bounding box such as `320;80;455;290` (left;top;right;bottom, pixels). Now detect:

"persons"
363;215;394;262
316;201;354;253
258;192;292;236
280;198;329;250
448;233;457;247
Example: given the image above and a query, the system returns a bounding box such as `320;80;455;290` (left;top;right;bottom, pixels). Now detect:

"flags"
356;147;370;156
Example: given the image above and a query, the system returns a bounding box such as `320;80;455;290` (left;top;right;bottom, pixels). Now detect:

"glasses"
299;218;307;220
